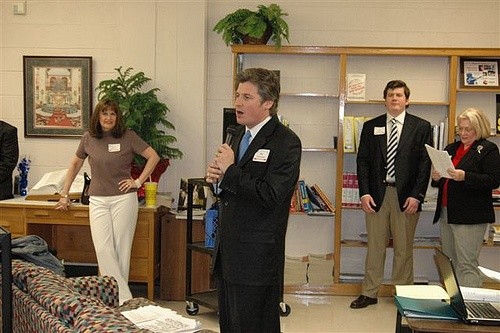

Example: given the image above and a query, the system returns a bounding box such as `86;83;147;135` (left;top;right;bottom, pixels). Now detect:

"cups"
143;182;158;205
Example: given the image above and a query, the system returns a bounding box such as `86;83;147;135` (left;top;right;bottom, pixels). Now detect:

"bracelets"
60;194;70;198
135;178;142;190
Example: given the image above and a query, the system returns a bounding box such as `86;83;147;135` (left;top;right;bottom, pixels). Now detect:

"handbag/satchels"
81;172;91;205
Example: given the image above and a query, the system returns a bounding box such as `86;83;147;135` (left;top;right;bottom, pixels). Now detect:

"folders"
393;295;461;320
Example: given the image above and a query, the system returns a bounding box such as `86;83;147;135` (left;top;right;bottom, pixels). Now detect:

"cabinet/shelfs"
230;44;500;295
186;177;218;311
0;195;161;301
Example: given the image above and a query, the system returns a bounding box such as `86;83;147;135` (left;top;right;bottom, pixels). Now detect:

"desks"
395;303;500;333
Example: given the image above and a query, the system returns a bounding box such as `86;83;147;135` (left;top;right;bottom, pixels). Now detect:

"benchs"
11;259;219;333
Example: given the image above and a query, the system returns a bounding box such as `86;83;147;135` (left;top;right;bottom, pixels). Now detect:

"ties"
236;130;252;166
385;119;398;183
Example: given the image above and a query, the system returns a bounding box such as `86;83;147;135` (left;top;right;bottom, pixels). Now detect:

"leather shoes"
349;295;377;309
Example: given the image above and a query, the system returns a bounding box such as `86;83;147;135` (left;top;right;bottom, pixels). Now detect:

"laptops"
433;247;500;326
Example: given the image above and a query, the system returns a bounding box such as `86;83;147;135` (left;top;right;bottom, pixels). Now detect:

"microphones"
226;124;236;145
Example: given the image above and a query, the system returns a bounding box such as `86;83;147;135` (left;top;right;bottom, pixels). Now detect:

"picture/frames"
23;55;92;137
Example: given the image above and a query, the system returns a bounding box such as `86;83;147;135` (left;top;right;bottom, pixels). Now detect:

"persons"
0;120;19;201
350;80;434;309
431;107;500;287
59;98;161;307
205;68;302;333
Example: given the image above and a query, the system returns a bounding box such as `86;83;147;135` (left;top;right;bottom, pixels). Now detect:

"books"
492;189;500;207
343;116;373;152
488;224;500;241
342;173;360;204
290;180;336;214
432;117;449;151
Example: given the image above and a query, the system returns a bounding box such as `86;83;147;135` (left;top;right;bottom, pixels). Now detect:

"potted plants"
213;3;290;52
95;67;182;195
